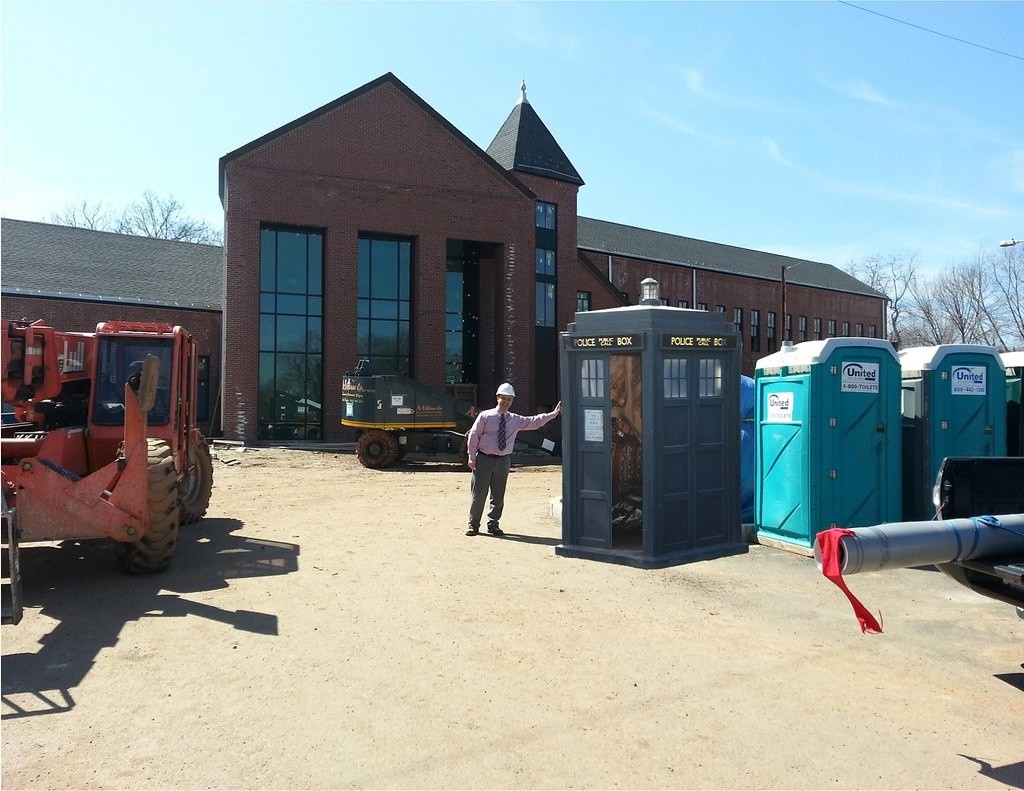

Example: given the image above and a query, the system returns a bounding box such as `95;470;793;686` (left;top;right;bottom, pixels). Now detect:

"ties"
498;413;507;450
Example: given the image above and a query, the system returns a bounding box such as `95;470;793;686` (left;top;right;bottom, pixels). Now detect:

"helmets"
496;383;515;397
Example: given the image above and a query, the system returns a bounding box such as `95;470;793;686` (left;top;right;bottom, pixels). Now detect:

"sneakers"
487;521;503;534
465;524;481;536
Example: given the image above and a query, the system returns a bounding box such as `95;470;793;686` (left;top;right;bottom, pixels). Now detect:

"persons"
465;382;561;535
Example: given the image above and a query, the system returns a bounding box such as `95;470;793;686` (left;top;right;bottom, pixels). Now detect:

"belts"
479;451;510;458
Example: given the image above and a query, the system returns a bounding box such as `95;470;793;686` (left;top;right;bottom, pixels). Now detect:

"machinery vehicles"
340;358;562;469
1;318;213;626
